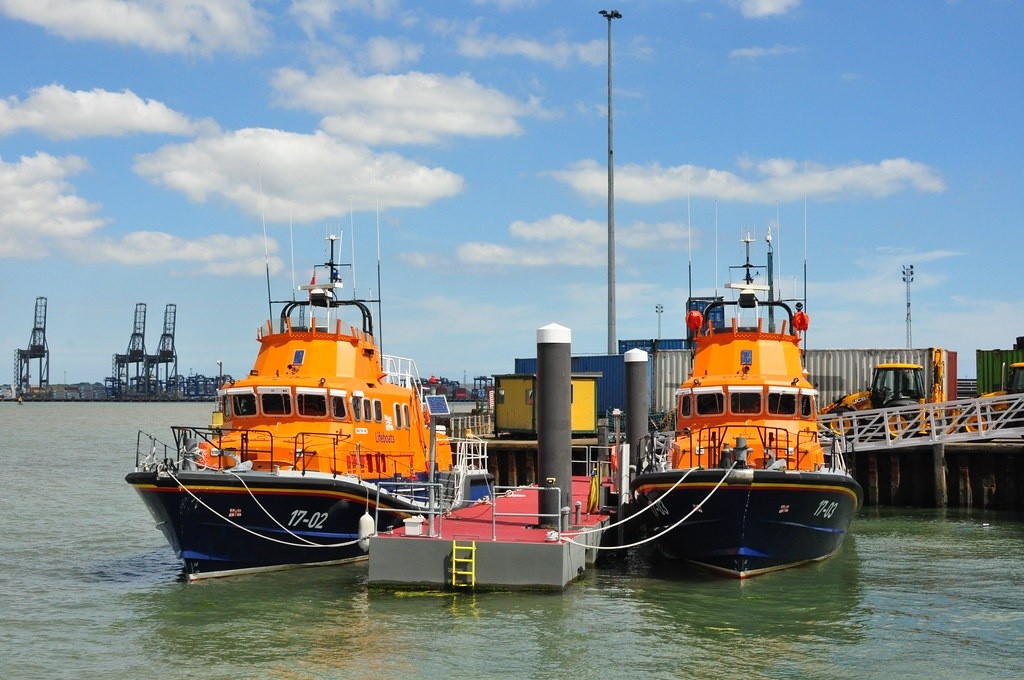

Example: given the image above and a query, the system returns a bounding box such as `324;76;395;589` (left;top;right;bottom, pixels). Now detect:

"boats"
125;197;495;580
630;196;862;579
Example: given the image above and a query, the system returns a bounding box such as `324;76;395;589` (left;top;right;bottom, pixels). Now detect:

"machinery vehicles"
946;362;1023;442
821;348;944;439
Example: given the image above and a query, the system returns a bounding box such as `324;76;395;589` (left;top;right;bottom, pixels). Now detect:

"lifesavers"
425;406;431;427
610;445;619;472
